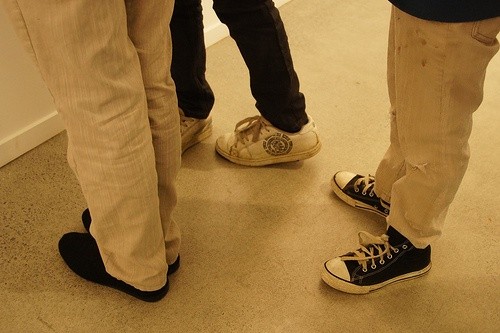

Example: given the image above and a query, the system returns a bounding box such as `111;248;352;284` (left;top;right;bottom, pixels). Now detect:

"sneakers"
179;109;322;167
321;170;433;295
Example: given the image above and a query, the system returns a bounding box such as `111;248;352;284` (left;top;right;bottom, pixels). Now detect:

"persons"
0;0;185;302
169;0;320;167
321;0;500;294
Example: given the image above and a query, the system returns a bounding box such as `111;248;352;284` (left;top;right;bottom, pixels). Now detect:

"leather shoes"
58;208;180;303
